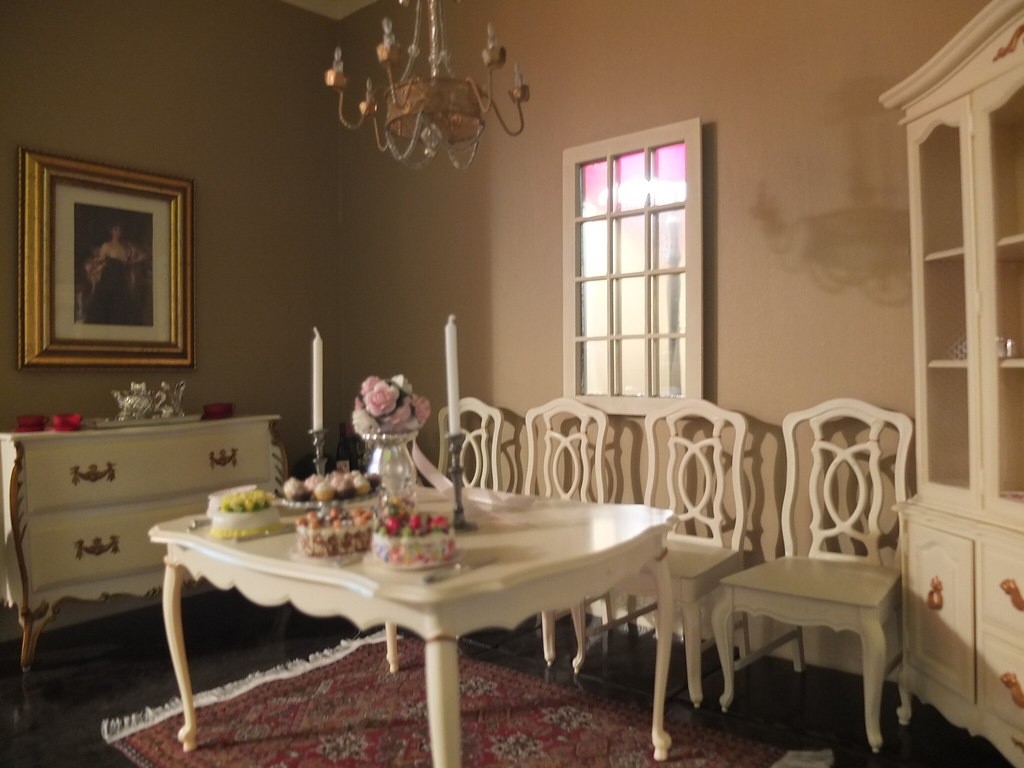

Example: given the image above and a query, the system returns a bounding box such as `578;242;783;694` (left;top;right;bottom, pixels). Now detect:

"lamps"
324;0;529;173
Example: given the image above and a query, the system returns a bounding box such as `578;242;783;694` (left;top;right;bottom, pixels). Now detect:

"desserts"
282;470;381;502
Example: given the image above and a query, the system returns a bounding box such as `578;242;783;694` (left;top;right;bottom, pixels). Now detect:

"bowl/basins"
296;508;373;557
214;504;279;531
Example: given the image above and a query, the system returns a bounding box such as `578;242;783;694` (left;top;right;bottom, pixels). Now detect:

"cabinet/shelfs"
878;0;1024;768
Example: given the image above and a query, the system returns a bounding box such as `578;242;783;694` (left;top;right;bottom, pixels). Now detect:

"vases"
370;431;419;499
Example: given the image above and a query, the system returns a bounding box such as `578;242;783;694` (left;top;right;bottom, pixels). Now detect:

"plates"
202;414;233;418
276;490;379;512
51;426;82;430
13;426;46;431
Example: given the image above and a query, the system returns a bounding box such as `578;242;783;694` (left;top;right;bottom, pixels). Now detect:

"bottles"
336;423;351;473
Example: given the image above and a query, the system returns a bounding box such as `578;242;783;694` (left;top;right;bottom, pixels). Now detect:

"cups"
204;402;236;415
16;415;49;427
52;413;84;427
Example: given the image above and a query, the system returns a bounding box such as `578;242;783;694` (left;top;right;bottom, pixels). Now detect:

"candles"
442;312;461;434
312;326;323;430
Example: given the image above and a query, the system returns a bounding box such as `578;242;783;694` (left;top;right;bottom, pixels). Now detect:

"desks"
0;411;290;673
149;472;678;767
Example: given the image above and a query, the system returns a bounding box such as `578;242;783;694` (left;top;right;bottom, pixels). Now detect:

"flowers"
352;373;431;437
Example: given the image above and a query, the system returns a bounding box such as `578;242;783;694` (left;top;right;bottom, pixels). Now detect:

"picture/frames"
19;146;198;374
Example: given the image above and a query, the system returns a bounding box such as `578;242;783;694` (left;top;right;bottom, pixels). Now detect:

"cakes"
205;490;284;538
294;506;459;570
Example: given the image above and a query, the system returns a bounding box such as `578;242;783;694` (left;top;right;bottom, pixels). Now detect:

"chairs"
438;393;505;490
513;394;608;674
621;399;753;706
713;398;914;753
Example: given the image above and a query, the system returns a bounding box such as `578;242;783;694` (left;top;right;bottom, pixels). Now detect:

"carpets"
100;624;833;767
